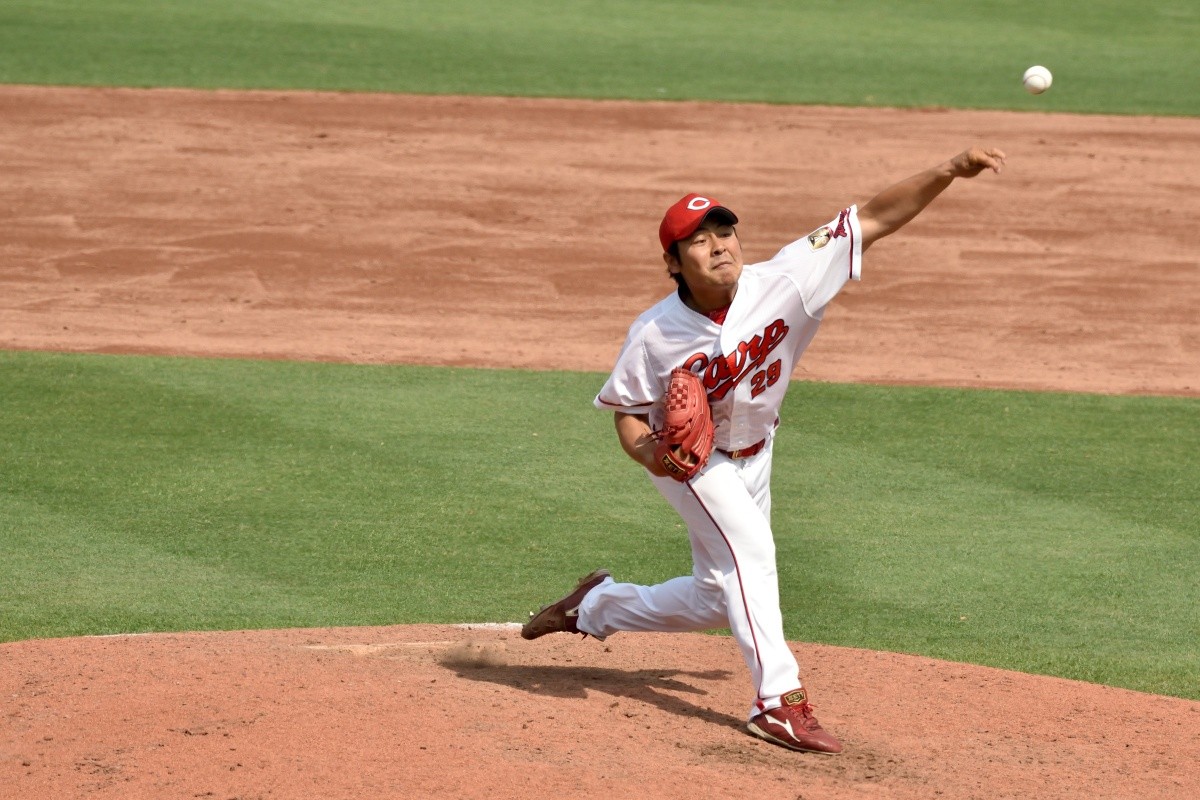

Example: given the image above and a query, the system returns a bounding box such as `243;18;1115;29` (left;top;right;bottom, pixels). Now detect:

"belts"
715;432;772;459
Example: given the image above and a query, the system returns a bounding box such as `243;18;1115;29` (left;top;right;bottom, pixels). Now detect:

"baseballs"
1023;65;1053;95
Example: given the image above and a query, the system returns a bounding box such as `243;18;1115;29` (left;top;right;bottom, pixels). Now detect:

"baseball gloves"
640;365;721;483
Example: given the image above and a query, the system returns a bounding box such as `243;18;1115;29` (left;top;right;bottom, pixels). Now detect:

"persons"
522;147;1006;754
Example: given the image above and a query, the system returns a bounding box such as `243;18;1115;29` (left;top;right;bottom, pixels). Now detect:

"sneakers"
521;569;610;640
747;687;843;755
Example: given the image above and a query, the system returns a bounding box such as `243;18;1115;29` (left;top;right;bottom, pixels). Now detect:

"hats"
659;193;739;251
